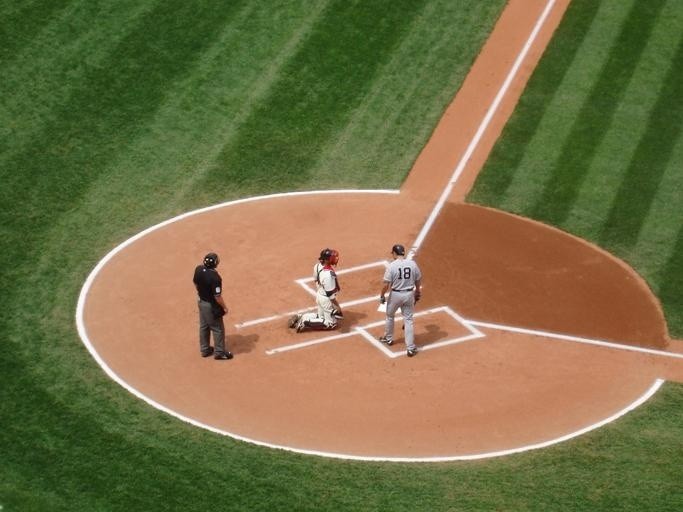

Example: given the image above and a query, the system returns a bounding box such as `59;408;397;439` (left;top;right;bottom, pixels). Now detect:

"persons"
192;251;233;360
376;243;423;357
286;248;345;333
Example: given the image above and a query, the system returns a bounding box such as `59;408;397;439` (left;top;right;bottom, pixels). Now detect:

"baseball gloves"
332;309;343;320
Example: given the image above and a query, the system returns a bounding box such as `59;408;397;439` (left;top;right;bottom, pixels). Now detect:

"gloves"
415;291;421;301
380;296;386;305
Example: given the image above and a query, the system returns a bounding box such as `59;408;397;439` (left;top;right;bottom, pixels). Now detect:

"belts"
391;288;414;293
199;297;210;303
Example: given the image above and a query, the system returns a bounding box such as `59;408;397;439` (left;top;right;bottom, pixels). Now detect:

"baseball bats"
402;292;420;330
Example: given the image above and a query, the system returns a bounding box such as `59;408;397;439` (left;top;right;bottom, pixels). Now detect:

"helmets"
390;244;405;255
319;249;338;265
203;253;219;269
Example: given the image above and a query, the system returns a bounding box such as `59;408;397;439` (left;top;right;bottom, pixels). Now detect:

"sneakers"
200;346;234;361
379;336;394;346
407;347;417;357
287;313;305;332
